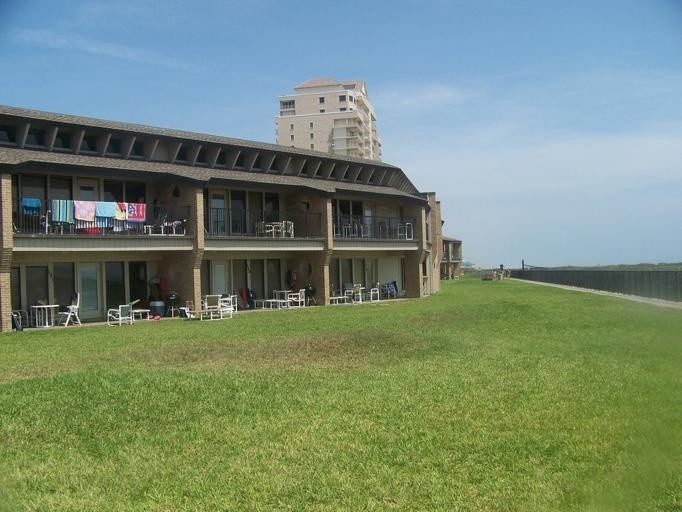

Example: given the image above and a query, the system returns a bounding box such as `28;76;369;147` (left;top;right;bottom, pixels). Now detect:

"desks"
30;304;61;327
129;310;151;321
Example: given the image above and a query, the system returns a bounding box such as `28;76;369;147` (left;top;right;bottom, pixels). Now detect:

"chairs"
253;289;306;309
187;294;238;320
329;282;380;304
333;223;369;238
39;210;64;235
11;305;29;328
255;220;294;238
144;219;188;235
56;289;82;326
107;298;141;326
